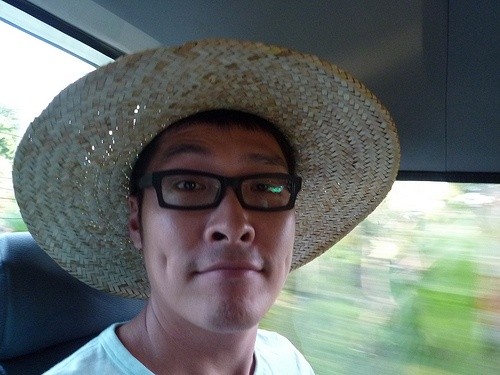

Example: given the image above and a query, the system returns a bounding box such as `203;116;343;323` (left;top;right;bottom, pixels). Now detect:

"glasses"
136;167;304;213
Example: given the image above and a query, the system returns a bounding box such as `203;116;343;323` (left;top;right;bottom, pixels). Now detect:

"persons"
12;38;401;375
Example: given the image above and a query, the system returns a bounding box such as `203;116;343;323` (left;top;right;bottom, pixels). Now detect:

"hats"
12;38;402;301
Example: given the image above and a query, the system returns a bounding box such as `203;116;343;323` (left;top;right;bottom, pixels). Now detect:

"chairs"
0;230;145;375
